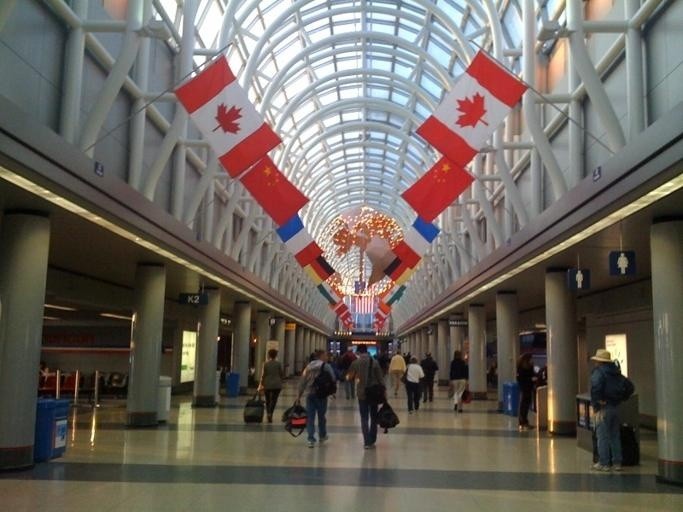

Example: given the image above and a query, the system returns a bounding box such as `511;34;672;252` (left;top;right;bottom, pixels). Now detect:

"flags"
282;215;444;333
170;50;283;183
401;159;497;224
411;51;537;165
240;156;314;230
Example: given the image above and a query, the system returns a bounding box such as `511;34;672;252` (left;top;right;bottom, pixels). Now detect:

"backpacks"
312;359;338;398
605;359;635;404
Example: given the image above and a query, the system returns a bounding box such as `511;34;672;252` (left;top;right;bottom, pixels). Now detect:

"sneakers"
593;460;624;473
407;398;465;414
520;424;537;431
305;437;377;450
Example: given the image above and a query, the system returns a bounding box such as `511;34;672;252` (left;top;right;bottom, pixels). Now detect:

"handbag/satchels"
460;383;473;403
281;398;308;430
364;381;388;407
376;400;402;430
400;367;408;384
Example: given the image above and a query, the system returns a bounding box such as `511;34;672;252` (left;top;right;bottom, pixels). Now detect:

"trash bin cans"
226;373;240;398
536;385;547;432
503;382;520;417
157;376;172;421
33;399;71;463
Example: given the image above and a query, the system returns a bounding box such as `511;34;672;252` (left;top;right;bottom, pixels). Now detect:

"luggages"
591;422;642;467
242;390;263;422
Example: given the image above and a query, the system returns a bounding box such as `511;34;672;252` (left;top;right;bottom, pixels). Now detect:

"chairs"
38;371;129;404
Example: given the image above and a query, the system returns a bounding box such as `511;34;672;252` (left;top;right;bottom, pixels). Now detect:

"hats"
589;348;615;364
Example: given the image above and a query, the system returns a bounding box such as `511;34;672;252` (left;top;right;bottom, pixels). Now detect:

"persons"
533;362;546;384
297;347;338;448
447;349;468;413
514;350;536;431
485;360;497;376
255;347;284;423
342;343;387;449
302;344;439;415
591;348;629;472
